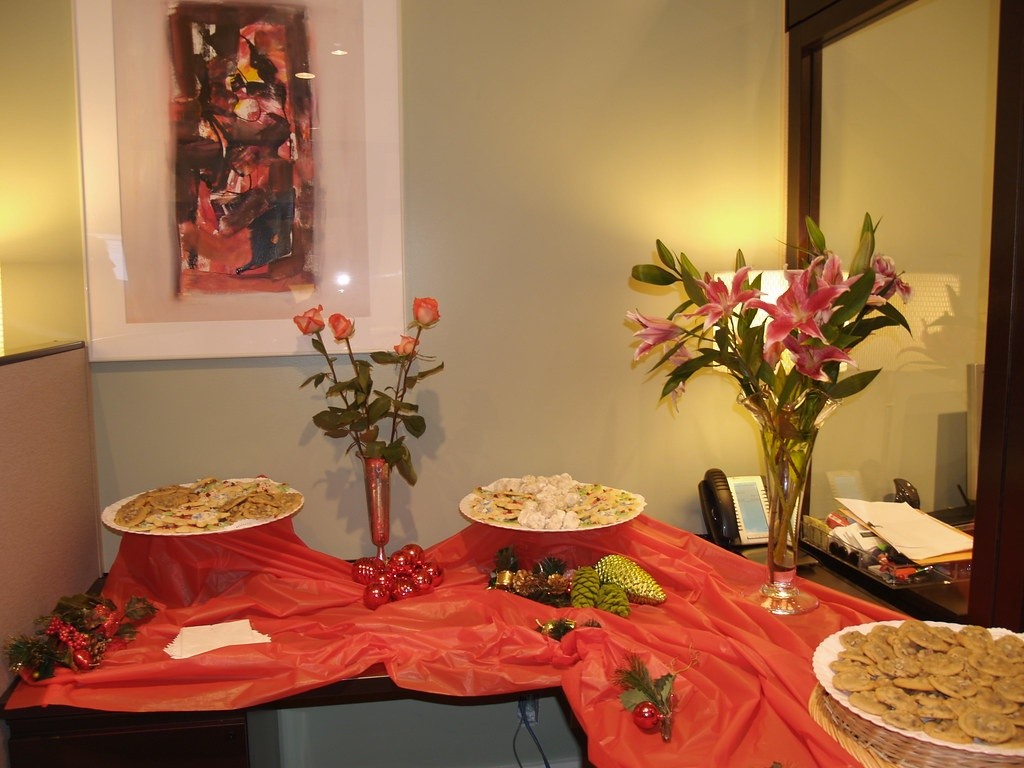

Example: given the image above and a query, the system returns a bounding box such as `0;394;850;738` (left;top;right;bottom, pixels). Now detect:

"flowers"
625;213;915;432
293;297;446;486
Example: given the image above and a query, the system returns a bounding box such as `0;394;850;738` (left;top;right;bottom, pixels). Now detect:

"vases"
747;427;821;615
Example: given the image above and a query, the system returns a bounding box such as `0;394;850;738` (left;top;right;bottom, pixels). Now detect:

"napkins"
162;618;272;660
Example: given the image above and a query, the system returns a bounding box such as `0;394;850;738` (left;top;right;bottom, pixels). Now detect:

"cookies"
828;620;1024;748
469;480;642;526
115;477;302;533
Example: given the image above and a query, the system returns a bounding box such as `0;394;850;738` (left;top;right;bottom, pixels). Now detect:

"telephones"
698;469;771;548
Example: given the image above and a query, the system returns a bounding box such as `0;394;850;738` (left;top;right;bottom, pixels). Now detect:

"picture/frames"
72;0;408;365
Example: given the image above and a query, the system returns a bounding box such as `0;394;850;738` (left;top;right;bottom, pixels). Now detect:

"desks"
798;504;975;625
0;535;915;768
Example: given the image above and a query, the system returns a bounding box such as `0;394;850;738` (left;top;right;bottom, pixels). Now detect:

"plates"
812;622;1024;758
459;478;645;532
101;478;304;536
